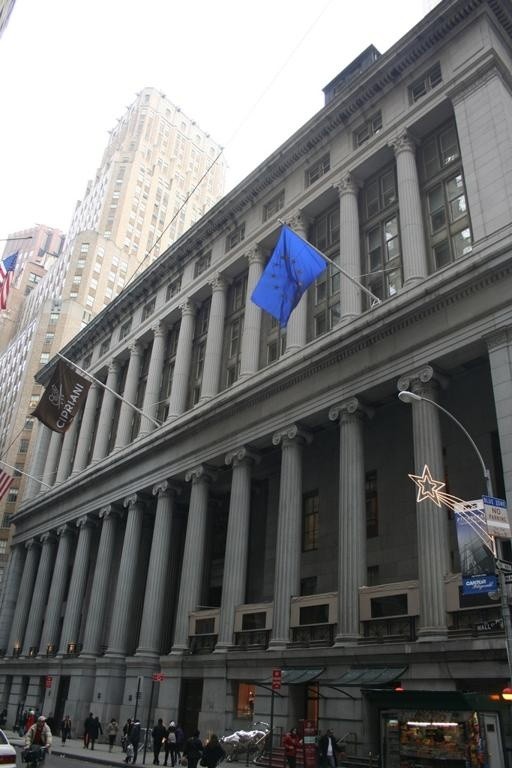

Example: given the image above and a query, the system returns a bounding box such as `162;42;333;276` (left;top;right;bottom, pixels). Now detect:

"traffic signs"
493;557;512;572
504;575;512;582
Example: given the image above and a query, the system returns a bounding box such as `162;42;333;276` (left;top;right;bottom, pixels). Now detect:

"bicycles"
21;741;51;768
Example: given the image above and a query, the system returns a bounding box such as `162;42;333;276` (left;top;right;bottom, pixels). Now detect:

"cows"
217;721;271;764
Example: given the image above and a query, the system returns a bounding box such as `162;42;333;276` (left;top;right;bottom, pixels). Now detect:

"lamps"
501;686;512;701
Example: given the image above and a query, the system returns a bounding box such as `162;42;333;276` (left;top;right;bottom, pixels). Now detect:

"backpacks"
166;730;177;744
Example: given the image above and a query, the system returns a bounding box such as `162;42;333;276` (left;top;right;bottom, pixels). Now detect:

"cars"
0;727;19;768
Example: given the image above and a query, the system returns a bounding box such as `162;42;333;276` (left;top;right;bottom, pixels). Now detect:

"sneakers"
60;742;181;768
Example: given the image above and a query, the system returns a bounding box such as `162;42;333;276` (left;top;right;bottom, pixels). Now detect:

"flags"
1;466;14;501
249;224;327;327
31;362;92;436
1;249;20;310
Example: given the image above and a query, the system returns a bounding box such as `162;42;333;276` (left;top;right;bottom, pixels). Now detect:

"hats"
169;721;176;728
37;716;48;721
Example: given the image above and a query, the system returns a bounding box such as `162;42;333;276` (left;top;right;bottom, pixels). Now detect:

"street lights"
395;388;512;665
